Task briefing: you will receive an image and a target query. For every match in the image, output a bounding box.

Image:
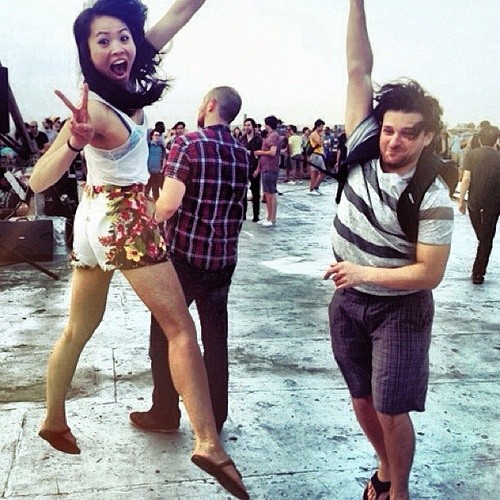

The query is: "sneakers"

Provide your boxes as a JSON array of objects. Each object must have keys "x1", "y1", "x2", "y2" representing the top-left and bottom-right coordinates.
[
  {"x1": 317, "y1": 190, "x2": 323, "y2": 195},
  {"x1": 299, "y1": 180, "x2": 304, "y2": 184},
  {"x1": 307, "y1": 189, "x2": 320, "y2": 196},
  {"x1": 257, "y1": 219, "x2": 268, "y2": 225},
  {"x1": 287, "y1": 181, "x2": 296, "y2": 185},
  {"x1": 262, "y1": 221, "x2": 276, "y2": 227}
]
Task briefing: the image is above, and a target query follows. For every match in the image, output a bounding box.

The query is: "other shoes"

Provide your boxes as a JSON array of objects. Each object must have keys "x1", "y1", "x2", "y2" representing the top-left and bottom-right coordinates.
[
  {"x1": 129, "y1": 412, "x2": 181, "y2": 432},
  {"x1": 473, "y1": 274, "x2": 483, "y2": 284}
]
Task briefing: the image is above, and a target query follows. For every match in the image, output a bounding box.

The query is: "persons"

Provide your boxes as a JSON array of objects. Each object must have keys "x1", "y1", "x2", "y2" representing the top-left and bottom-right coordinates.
[
  {"x1": 131, "y1": 87, "x2": 251, "y2": 435},
  {"x1": 458, "y1": 125, "x2": 499, "y2": 288},
  {"x1": 323, "y1": 1, "x2": 455, "y2": 500},
  {"x1": 0, "y1": 116, "x2": 499, "y2": 222},
  {"x1": 28, "y1": 1, "x2": 250, "y2": 500}
]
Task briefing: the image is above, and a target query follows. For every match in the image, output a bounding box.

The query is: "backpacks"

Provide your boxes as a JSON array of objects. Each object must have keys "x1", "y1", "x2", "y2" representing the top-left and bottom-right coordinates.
[{"x1": 335, "y1": 134, "x2": 459, "y2": 243}]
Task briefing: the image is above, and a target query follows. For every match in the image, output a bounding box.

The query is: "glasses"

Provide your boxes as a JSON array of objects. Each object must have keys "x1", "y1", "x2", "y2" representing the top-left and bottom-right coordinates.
[{"x1": 29, "y1": 126, "x2": 34, "y2": 128}]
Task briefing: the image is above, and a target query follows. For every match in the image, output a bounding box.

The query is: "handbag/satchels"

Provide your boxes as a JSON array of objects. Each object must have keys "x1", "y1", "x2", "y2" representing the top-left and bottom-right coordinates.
[{"x1": 307, "y1": 145, "x2": 319, "y2": 155}]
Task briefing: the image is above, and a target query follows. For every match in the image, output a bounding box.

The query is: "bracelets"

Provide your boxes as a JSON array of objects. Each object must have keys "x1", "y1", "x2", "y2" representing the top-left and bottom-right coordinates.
[{"x1": 65, "y1": 136, "x2": 87, "y2": 158}]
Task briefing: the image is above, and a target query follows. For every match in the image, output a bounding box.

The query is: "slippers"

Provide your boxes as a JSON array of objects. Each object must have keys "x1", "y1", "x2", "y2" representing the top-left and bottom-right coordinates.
[
  {"x1": 39, "y1": 428, "x2": 80, "y2": 453},
  {"x1": 192, "y1": 454, "x2": 250, "y2": 500},
  {"x1": 362, "y1": 472, "x2": 391, "y2": 500}
]
